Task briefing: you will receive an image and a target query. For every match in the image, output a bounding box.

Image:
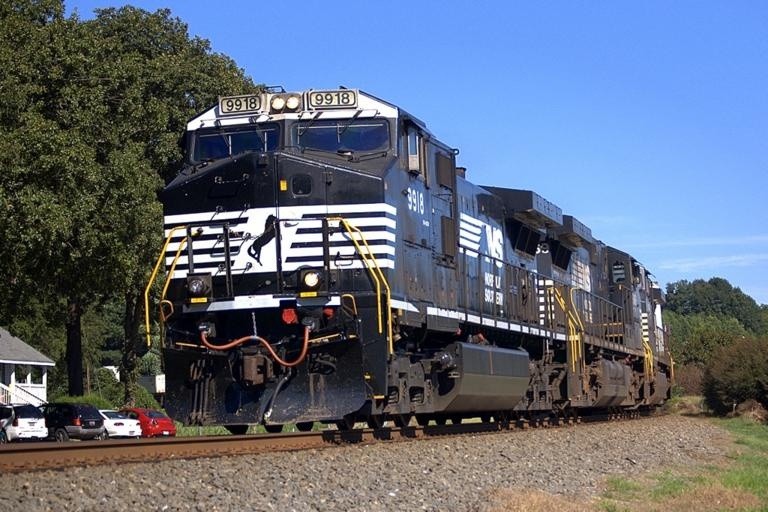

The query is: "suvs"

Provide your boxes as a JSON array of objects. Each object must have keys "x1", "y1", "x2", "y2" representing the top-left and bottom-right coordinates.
[{"x1": 0, "y1": 401, "x2": 106, "y2": 444}]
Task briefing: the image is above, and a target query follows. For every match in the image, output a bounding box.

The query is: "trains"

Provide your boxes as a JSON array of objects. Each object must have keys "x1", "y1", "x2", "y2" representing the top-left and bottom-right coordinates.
[{"x1": 158, "y1": 84, "x2": 676, "y2": 434}]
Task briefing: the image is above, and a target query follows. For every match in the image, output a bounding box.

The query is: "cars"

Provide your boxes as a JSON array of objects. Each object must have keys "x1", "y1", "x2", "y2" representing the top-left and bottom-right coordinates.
[{"x1": 89, "y1": 407, "x2": 177, "y2": 440}]
